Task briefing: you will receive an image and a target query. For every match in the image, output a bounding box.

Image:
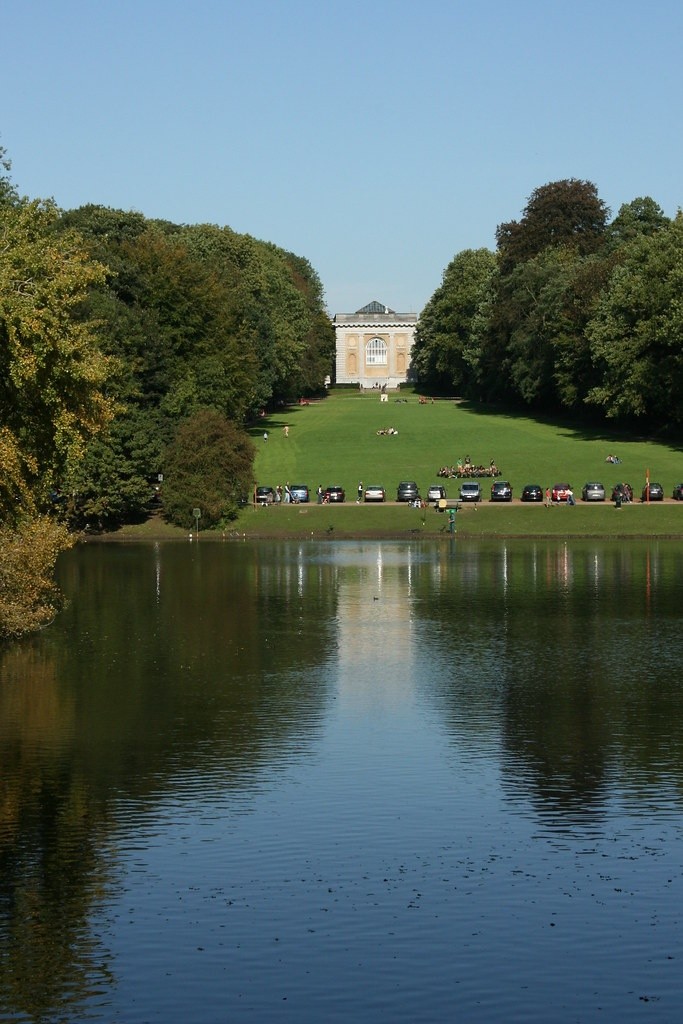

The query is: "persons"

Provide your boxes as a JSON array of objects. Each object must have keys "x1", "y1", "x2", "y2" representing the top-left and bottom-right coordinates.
[
  {"x1": 356, "y1": 482, "x2": 363, "y2": 504},
  {"x1": 556, "y1": 493, "x2": 561, "y2": 503},
  {"x1": 565, "y1": 489, "x2": 575, "y2": 505},
  {"x1": 263, "y1": 433, "x2": 268, "y2": 442},
  {"x1": 395, "y1": 398, "x2": 408, "y2": 403},
  {"x1": 438, "y1": 455, "x2": 502, "y2": 479},
  {"x1": 419, "y1": 396, "x2": 434, "y2": 404},
  {"x1": 545, "y1": 488, "x2": 553, "y2": 507},
  {"x1": 266, "y1": 481, "x2": 300, "y2": 504},
  {"x1": 300, "y1": 396, "x2": 309, "y2": 406},
  {"x1": 377, "y1": 427, "x2": 398, "y2": 436},
  {"x1": 316, "y1": 485, "x2": 327, "y2": 504},
  {"x1": 282, "y1": 426, "x2": 289, "y2": 439},
  {"x1": 420, "y1": 500, "x2": 426, "y2": 521},
  {"x1": 616, "y1": 483, "x2": 631, "y2": 509},
  {"x1": 606, "y1": 455, "x2": 620, "y2": 464},
  {"x1": 448, "y1": 509, "x2": 455, "y2": 535}
]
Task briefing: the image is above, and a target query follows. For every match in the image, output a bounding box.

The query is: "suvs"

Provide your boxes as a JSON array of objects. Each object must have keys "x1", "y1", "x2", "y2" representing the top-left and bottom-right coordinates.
[
  {"x1": 326, "y1": 487, "x2": 344, "y2": 502},
  {"x1": 491, "y1": 482, "x2": 511, "y2": 501},
  {"x1": 552, "y1": 483, "x2": 571, "y2": 501},
  {"x1": 642, "y1": 483, "x2": 663, "y2": 501}
]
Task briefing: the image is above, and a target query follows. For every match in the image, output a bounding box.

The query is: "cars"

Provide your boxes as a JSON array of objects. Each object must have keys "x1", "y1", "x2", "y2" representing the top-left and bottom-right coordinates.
[
  {"x1": 673, "y1": 484, "x2": 683, "y2": 501},
  {"x1": 365, "y1": 485, "x2": 384, "y2": 502},
  {"x1": 254, "y1": 486, "x2": 276, "y2": 502},
  {"x1": 428, "y1": 486, "x2": 445, "y2": 501},
  {"x1": 523, "y1": 485, "x2": 543, "y2": 501},
  {"x1": 612, "y1": 483, "x2": 633, "y2": 501}
]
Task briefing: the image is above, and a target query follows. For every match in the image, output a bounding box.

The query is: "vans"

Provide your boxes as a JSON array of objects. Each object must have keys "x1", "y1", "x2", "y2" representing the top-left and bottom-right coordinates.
[
  {"x1": 583, "y1": 483, "x2": 604, "y2": 501},
  {"x1": 398, "y1": 481, "x2": 418, "y2": 501},
  {"x1": 289, "y1": 485, "x2": 308, "y2": 502},
  {"x1": 460, "y1": 482, "x2": 480, "y2": 501}
]
